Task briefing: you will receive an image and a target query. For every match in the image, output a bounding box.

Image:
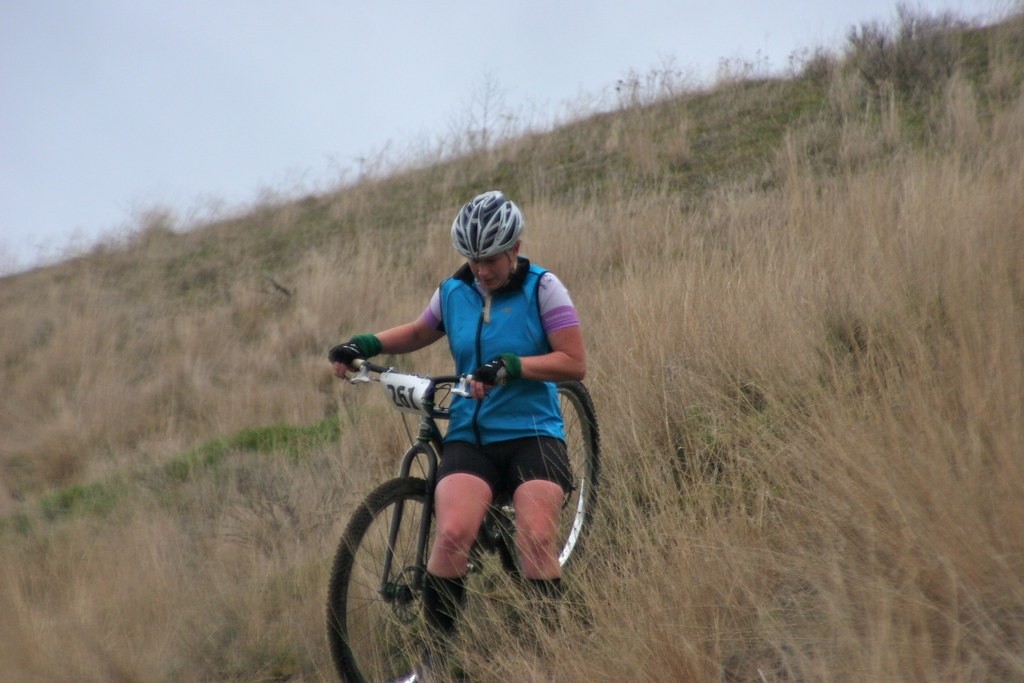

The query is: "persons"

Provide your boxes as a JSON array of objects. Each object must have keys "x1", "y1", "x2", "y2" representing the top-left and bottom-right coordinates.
[{"x1": 328, "y1": 189, "x2": 587, "y2": 683}]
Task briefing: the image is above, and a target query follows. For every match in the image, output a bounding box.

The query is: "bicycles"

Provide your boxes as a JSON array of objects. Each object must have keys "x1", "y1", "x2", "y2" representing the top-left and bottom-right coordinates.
[{"x1": 326, "y1": 358, "x2": 601, "y2": 683}]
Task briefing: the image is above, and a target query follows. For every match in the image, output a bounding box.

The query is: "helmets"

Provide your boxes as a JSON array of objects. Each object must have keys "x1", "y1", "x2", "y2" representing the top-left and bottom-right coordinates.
[{"x1": 451, "y1": 190, "x2": 523, "y2": 260}]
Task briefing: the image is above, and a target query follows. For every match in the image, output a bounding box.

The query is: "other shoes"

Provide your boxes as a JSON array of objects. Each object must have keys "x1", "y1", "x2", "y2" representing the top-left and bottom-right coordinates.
[{"x1": 391, "y1": 664, "x2": 445, "y2": 683}]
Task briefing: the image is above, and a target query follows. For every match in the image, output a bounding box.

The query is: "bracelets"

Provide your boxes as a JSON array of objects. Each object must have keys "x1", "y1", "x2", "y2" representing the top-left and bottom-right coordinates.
[
  {"x1": 349, "y1": 333, "x2": 382, "y2": 358},
  {"x1": 500, "y1": 353, "x2": 521, "y2": 381}
]
